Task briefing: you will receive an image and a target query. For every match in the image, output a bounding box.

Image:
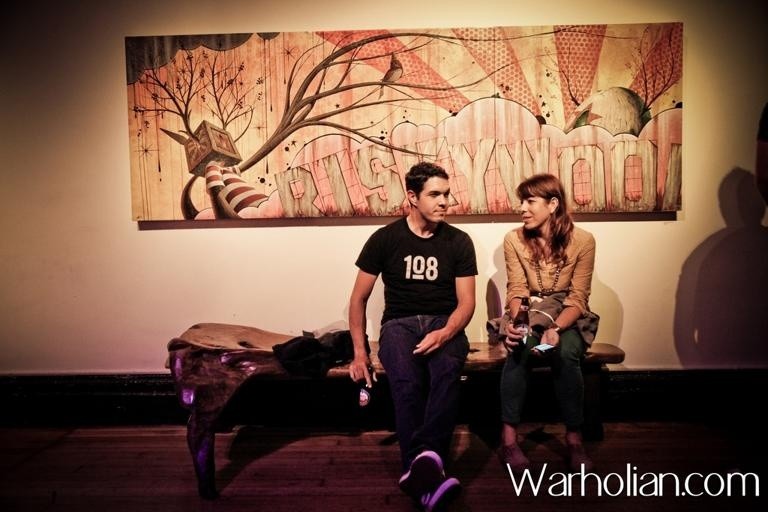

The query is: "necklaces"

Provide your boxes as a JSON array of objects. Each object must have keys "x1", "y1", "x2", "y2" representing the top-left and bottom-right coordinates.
[{"x1": 533, "y1": 255, "x2": 561, "y2": 293}]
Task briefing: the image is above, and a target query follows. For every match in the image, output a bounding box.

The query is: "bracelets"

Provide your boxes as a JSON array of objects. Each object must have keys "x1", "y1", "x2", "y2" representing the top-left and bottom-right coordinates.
[{"x1": 548, "y1": 323, "x2": 562, "y2": 335}]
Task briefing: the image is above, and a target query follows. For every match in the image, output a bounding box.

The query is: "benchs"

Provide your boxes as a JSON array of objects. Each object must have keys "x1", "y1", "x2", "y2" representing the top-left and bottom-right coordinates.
[{"x1": 167, "y1": 321, "x2": 625, "y2": 501}]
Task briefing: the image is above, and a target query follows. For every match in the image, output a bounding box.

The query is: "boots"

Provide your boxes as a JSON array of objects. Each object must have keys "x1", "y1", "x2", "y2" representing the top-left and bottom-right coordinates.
[
  {"x1": 568, "y1": 443, "x2": 593, "y2": 471},
  {"x1": 496, "y1": 441, "x2": 531, "y2": 473}
]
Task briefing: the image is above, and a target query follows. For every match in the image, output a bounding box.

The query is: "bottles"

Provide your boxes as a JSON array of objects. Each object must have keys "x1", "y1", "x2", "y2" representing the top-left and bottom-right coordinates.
[
  {"x1": 510, "y1": 297, "x2": 530, "y2": 365},
  {"x1": 359, "y1": 363, "x2": 375, "y2": 409}
]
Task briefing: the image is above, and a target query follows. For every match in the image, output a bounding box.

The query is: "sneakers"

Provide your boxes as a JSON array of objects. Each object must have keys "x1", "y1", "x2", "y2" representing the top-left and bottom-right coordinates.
[{"x1": 397, "y1": 451, "x2": 460, "y2": 512}]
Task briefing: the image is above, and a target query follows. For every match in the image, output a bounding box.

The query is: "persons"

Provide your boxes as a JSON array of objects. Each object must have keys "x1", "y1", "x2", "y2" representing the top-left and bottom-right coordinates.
[
  {"x1": 497, "y1": 173, "x2": 605, "y2": 486},
  {"x1": 347, "y1": 162, "x2": 479, "y2": 509}
]
